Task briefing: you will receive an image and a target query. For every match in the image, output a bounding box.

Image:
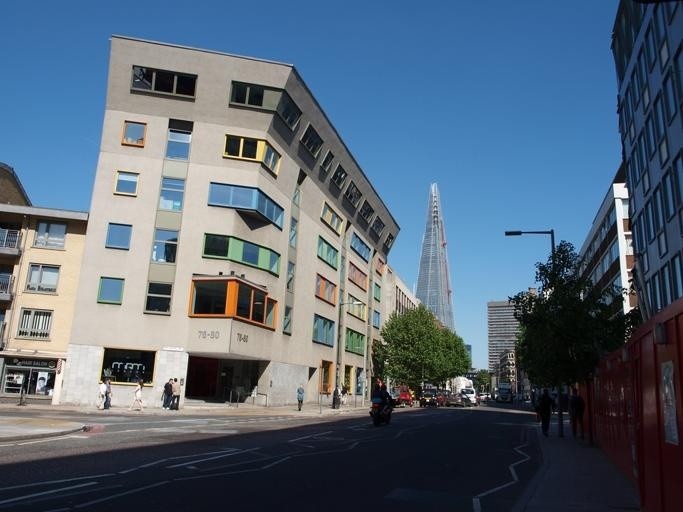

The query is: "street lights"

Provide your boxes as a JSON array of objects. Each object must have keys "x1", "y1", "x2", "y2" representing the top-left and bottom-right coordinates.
[
  {"x1": 332, "y1": 301, "x2": 364, "y2": 409},
  {"x1": 504, "y1": 226, "x2": 566, "y2": 436}
]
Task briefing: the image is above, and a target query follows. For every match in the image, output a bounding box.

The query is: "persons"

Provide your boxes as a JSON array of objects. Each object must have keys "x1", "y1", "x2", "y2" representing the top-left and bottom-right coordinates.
[
  {"x1": 334, "y1": 384, "x2": 341, "y2": 409},
  {"x1": 537, "y1": 389, "x2": 556, "y2": 438},
  {"x1": 169, "y1": 378, "x2": 181, "y2": 410},
  {"x1": 372, "y1": 382, "x2": 392, "y2": 414},
  {"x1": 36, "y1": 377, "x2": 47, "y2": 396},
  {"x1": 567, "y1": 388, "x2": 585, "y2": 439},
  {"x1": 340, "y1": 385, "x2": 349, "y2": 405},
  {"x1": 129, "y1": 380, "x2": 146, "y2": 412},
  {"x1": 160, "y1": 378, "x2": 174, "y2": 410},
  {"x1": 95, "y1": 376, "x2": 114, "y2": 410},
  {"x1": 297, "y1": 383, "x2": 304, "y2": 411}
]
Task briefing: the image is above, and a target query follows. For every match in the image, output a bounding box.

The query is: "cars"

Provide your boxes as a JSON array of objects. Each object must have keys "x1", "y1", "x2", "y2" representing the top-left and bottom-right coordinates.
[{"x1": 391, "y1": 383, "x2": 530, "y2": 410}]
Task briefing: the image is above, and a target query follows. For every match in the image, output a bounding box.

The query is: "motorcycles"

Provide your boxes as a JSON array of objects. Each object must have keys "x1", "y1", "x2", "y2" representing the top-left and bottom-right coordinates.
[{"x1": 369, "y1": 397, "x2": 394, "y2": 425}]
